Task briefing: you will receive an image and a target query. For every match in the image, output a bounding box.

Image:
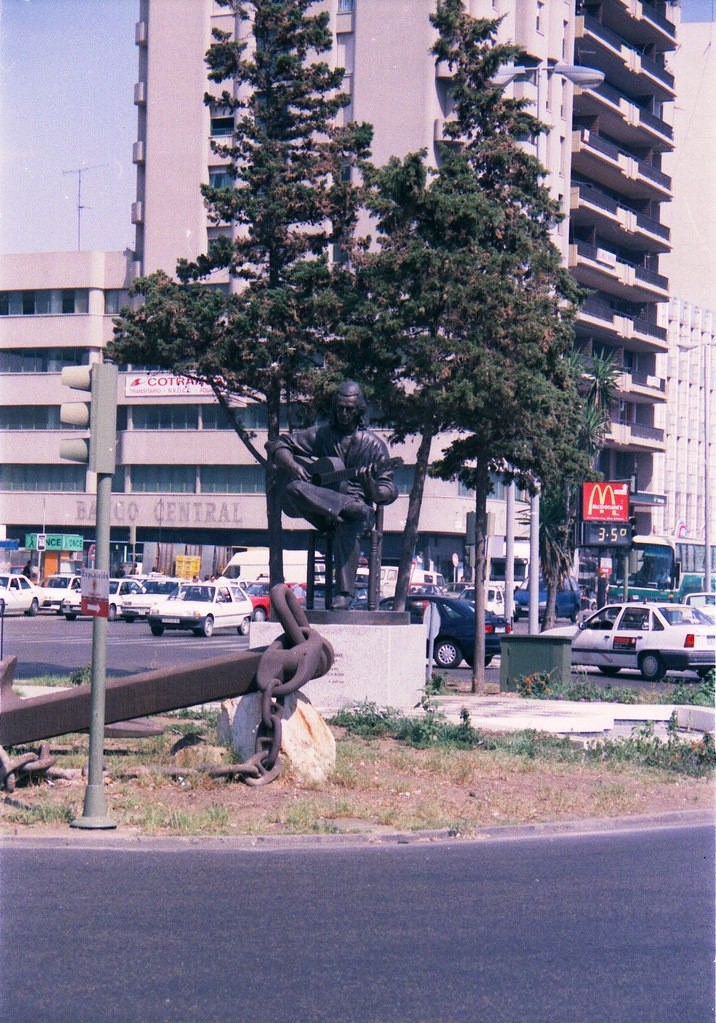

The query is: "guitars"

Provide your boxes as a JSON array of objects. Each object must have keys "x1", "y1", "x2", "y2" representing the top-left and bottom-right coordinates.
[{"x1": 277, "y1": 455, "x2": 405, "y2": 519}]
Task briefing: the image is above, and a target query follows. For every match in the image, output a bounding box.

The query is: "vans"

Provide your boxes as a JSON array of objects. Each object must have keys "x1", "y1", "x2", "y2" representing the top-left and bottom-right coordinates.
[{"x1": 220, "y1": 548, "x2": 326, "y2": 584}]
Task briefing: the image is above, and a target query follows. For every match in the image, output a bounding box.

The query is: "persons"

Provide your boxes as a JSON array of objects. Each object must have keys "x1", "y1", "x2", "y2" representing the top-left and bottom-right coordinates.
[
  {"x1": 580, "y1": 586, "x2": 594, "y2": 610},
  {"x1": 149, "y1": 567, "x2": 158, "y2": 577},
  {"x1": 460, "y1": 577, "x2": 465, "y2": 582},
  {"x1": 264, "y1": 382, "x2": 399, "y2": 610},
  {"x1": 76, "y1": 563, "x2": 86, "y2": 576},
  {"x1": 190, "y1": 573, "x2": 230, "y2": 601},
  {"x1": 23, "y1": 561, "x2": 31, "y2": 579},
  {"x1": 128, "y1": 563, "x2": 139, "y2": 575},
  {"x1": 656, "y1": 570, "x2": 670, "y2": 584},
  {"x1": 114, "y1": 567, "x2": 125, "y2": 578}
]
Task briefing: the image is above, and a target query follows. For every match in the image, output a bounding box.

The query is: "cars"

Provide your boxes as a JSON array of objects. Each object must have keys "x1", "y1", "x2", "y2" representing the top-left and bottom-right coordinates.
[
  {"x1": 147, "y1": 582, "x2": 253, "y2": 637},
  {"x1": 309, "y1": 581, "x2": 383, "y2": 611},
  {"x1": 680, "y1": 592, "x2": 716, "y2": 620},
  {"x1": 0, "y1": 574, "x2": 45, "y2": 618},
  {"x1": 63, "y1": 572, "x2": 203, "y2": 623},
  {"x1": 539, "y1": 599, "x2": 716, "y2": 682},
  {"x1": 341, "y1": 563, "x2": 587, "y2": 669},
  {"x1": 121, "y1": 578, "x2": 187, "y2": 624},
  {"x1": 244, "y1": 581, "x2": 309, "y2": 623},
  {"x1": 36, "y1": 572, "x2": 78, "y2": 617}
]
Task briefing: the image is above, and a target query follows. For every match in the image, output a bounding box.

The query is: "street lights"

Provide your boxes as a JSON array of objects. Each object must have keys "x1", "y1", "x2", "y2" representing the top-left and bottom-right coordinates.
[{"x1": 677, "y1": 340, "x2": 716, "y2": 592}]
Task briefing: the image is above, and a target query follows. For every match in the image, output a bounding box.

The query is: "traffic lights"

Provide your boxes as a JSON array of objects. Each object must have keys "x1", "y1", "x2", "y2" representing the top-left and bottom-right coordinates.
[{"x1": 58, "y1": 359, "x2": 117, "y2": 475}]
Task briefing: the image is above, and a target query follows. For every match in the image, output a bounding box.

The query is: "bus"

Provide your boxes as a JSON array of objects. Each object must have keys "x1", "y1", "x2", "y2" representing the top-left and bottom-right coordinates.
[{"x1": 603, "y1": 535, "x2": 716, "y2": 612}]
